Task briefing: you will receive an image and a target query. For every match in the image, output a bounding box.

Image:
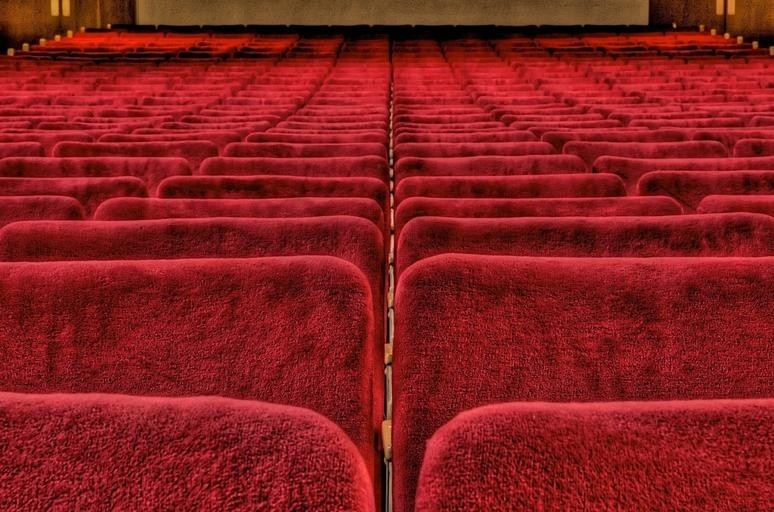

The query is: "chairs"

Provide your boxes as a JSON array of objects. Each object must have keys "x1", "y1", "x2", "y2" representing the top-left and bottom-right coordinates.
[{"x1": 0, "y1": 22, "x2": 773, "y2": 512}]
